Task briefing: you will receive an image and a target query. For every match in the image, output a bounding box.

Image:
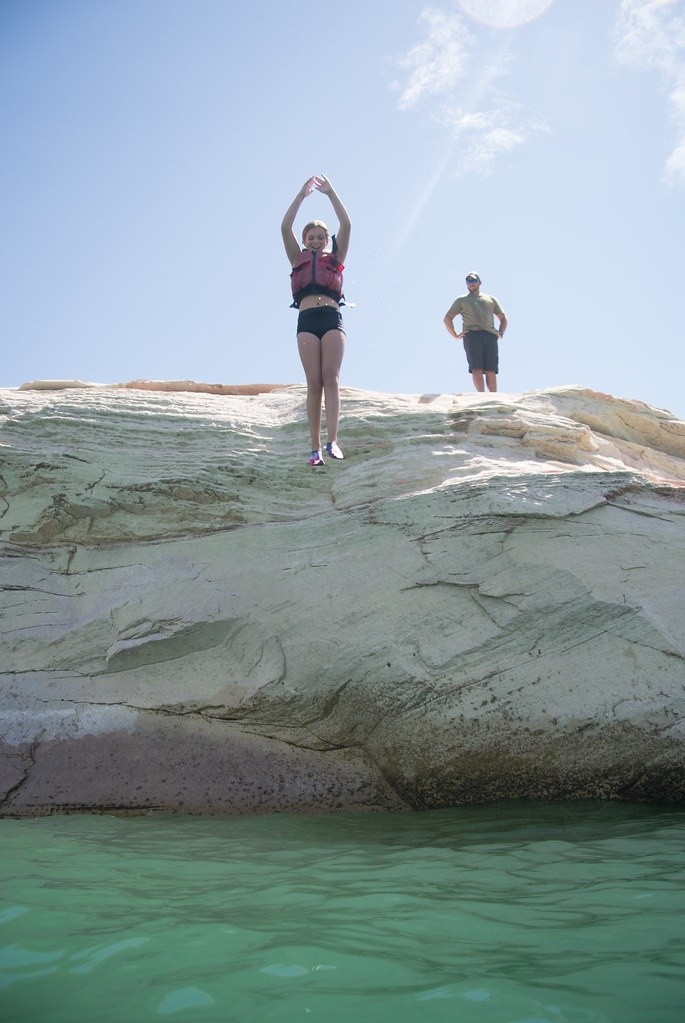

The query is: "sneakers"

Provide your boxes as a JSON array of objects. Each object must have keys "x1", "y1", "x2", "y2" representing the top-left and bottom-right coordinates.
[
  {"x1": 310, "y1": 450, "x2": 324, "y2": 465},
  {"x1": 327, "y1": 443, "x2": 344, "y2": 460}
]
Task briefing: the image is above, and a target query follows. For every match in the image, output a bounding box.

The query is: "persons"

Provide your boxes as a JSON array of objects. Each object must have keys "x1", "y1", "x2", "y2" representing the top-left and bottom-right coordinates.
[
  {"x1": 281, "y1": 172, "x2": 352, "y2": 470},
  {"x1": 444, "y1": 271, "x2": 507, "y2": 392}
]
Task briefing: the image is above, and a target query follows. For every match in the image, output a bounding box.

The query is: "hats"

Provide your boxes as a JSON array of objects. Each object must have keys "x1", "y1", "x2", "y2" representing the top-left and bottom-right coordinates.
[{"x1": 466, "y1": 273, "x2": 481, "y2": 283}]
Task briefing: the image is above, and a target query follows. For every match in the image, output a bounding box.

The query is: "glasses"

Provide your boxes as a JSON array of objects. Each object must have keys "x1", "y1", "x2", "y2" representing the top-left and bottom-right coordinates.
[{"x1": 466, "y1": 279, "x2": 478, "y2": 284}]
